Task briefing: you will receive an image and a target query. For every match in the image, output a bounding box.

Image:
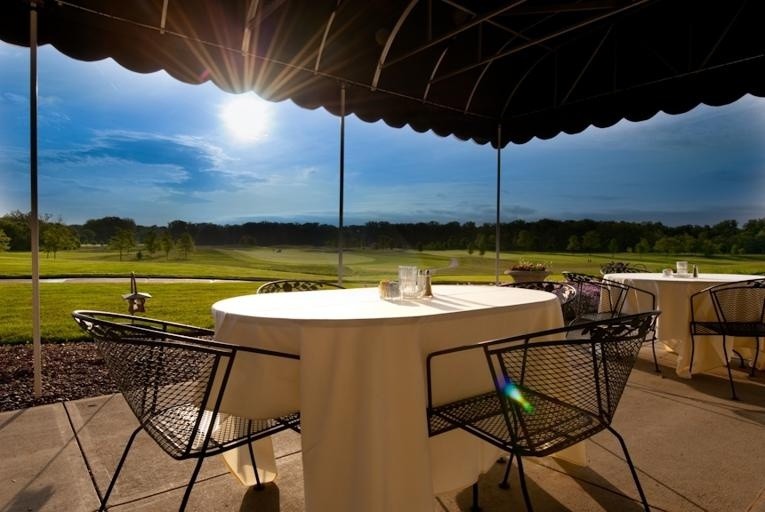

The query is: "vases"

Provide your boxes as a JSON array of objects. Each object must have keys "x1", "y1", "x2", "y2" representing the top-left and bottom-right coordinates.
[{"x1": 507, "y1": 267, "x2": 553, "y2": 282}]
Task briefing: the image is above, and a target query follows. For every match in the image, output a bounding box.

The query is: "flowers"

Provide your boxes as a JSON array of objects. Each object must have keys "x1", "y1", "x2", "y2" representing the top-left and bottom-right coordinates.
[{"x1": 509, "y1": 257, "x2": 555, "y2": 272}]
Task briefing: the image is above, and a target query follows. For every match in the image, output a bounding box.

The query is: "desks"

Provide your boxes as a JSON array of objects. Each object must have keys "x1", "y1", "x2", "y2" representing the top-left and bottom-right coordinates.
[
  {"x1": 207, "y1": 285, "x2": 565, "y2": 512},
  {"x1": 598, "y1": 272, "x2": 765, "y2": 378}
]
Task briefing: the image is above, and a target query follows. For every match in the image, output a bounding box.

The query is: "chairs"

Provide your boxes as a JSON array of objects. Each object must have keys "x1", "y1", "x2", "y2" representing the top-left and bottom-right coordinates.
[
  {"x1": 683, "y1": 278, "x2": 765, "y2": 402},
  {"x1": 564, "y1": 270, "x2": 662, "y2": 373},
  {"x1": 599, "y1": 265, "x2": 640, "y2": 276},
  {"x1": 504, "y1": 280, "x2": 579, "y2": 306},
  {"x1": 64, "y1": 304, "x2": 301, "y2": 509},
  {"x1": 422, "y1": 309, "x2": 660, "y2": 510},
  {"x1": 250, "y1": 279, "x2": 351, "y2": 491}
]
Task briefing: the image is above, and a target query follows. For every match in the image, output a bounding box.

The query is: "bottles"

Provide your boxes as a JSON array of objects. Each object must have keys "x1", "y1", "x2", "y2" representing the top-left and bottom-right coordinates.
[
  {"x1": 693, "y1": 264, "x2": 698, "y2": 277},
  {"x1": 416, "y1": 269, "x2": 425, "y2": 298},
  {"x1": 422, "y1": 270, "x2": 433, "y2": 299}
]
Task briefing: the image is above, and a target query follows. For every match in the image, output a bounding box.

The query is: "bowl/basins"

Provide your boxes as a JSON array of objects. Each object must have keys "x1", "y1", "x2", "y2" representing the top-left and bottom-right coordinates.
[{"x1": 662, "y1": 269, "x2": 674, "y2": 276}]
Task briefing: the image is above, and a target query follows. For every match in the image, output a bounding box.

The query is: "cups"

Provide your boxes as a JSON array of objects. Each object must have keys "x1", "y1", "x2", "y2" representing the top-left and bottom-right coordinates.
[
  {"x1": 676, "y1": 261, "x2": 687, "y2": 277},
  {"x1": 398, "y1": 265, "x2": 418, "y2": 299}
]
